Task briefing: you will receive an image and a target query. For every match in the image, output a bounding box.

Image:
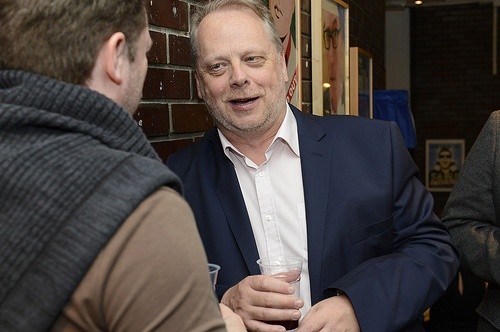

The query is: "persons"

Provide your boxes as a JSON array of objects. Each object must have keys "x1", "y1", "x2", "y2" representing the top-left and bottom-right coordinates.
[
  {"x1": 159, "y1": 0, "x2": 460, "y2": 332},
  {"x1": 443, "y1": 110, "x2": 500, "y2": 331},
  {"x1": 320, "y1": 11, "x2": 346, "y2": 115},
  {"x1": 0, "y1": 1, "x2": 247, "y2": 332}
]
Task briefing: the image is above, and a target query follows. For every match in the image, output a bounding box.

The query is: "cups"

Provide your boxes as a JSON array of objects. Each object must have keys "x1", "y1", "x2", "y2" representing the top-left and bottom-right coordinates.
[
  {"x1": 257, "y1": 256, "x2": 304, "y2": 300},
  {"x1": 208, "y1": 261, "x2": 220, "y2": 294}
]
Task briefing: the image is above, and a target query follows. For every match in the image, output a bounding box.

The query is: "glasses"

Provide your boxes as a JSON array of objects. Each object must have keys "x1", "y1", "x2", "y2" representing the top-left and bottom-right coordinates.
[{"x1": 438, "y1": 155, "x2": 451, "y2": 159}]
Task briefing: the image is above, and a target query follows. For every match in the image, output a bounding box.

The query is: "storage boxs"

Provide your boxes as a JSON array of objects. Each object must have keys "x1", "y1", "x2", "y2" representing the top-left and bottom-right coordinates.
[{"x1": 424, "y1": 139, "x2": 465, "y2": 193}]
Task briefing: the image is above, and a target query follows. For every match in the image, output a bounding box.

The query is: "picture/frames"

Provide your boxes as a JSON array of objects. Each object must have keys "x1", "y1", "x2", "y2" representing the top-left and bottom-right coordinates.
[
  {"x1": 261, "y1": 0, "x2": 303, "y2": 111},
  {"x1": 349, "y1": 47, "x2": 373, "y2": 119},
  {"x1": 311, "y1": 0, "x2": 350, "y2": 117}
]
